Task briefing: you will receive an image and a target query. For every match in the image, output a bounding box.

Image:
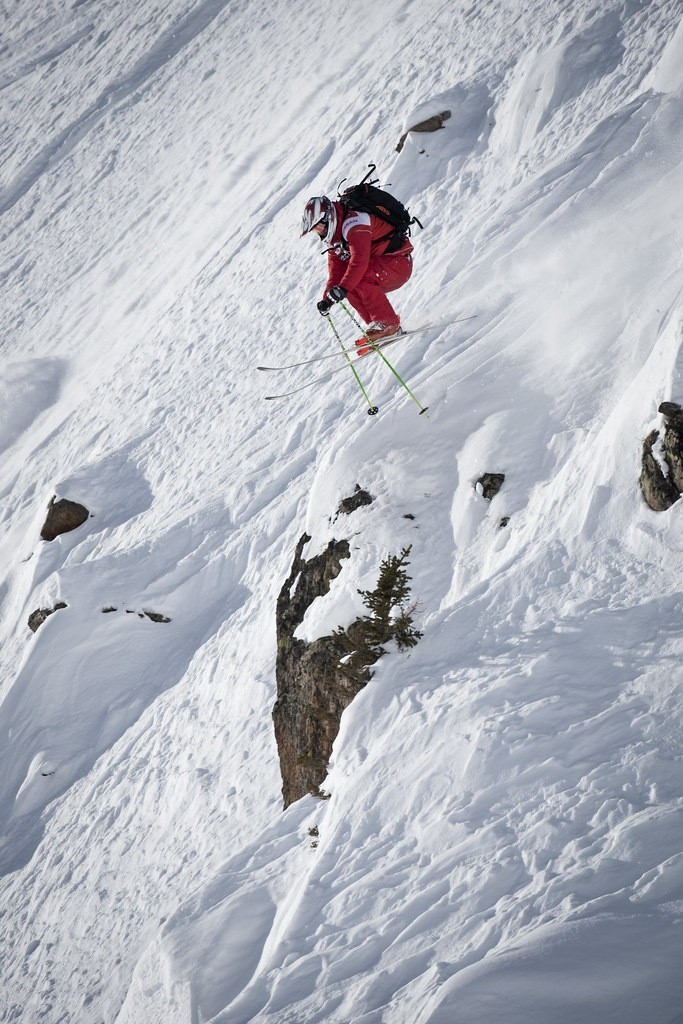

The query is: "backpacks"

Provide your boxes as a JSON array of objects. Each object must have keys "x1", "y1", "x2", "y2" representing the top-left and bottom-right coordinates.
[{"x1": 340, "y1": 184, "x2": 411, "y2": 238}]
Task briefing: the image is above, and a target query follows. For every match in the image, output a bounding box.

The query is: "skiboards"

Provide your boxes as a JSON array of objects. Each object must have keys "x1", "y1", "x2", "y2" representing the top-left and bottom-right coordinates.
[{"x1": 256, "y1": 315, "x2": 478, "y2": 401}]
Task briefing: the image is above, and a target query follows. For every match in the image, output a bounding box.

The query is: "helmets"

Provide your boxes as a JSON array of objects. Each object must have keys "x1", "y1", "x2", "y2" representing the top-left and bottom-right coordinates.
[{"x1": 300, "y1": 196, "x2": 330, "y2": 237}]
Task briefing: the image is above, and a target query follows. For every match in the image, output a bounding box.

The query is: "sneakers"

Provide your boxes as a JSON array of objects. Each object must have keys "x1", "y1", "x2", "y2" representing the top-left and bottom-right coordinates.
[{"x1": 354, "y1": 318, "x2": 404, "y2": 358}]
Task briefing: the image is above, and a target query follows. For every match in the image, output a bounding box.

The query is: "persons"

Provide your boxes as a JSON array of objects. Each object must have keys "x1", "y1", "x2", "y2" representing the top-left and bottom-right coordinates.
[{"x1": 295, "y1": 193, "x2": 416, "y2": 355}]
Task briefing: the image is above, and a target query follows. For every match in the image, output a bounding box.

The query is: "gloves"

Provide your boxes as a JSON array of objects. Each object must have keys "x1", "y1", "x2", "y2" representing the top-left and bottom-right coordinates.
[
  {"x1": 327, "y1": 285, "x2": 347, "y2": 304},
  {"x1": 317, "y1": 299, "x2": 333, "y2": 316}
]
